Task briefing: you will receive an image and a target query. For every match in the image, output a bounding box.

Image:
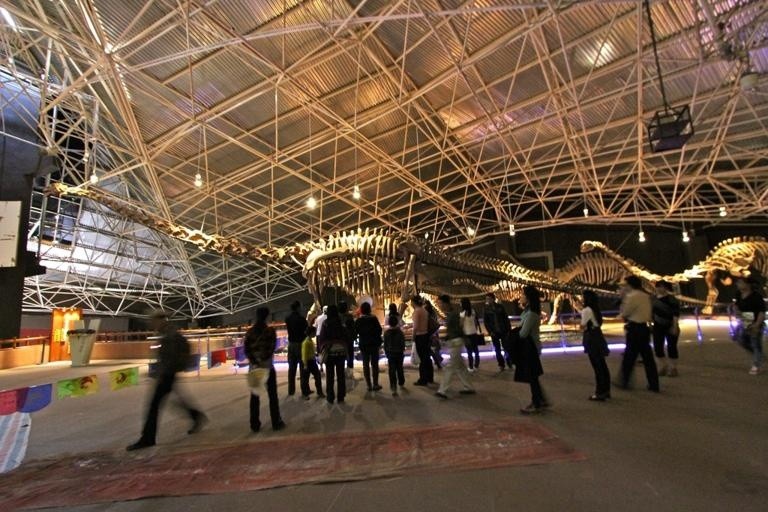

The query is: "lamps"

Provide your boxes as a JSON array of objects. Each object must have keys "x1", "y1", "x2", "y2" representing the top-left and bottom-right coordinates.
[
  {"x1": 192, "y1": 122, "x2": 209, "y2": 189},
  {"x1": 305, "y1": 109, "x2": 317, "y2": 211},
  {"x1": 351, "y1": 41, "x2": 363, "y2": 202},
  {"x1": 679, "y1": 208, "x2": 690, "y2": 242},
  {"x1": 636, "y1": 193, "x2": 647, "y2": 245},
  {"x1": 463, "y1": 216, "x2": 477, "y2": 239},
  {"x1": 500, "y1": 131, "x2": 516, "y2": 239},
  {"x1": 87, "y1": 96, "x2": 100, "y2": 186},
  {"x1": 581, "y1": 158, "x2": 590, "y2": 219}
]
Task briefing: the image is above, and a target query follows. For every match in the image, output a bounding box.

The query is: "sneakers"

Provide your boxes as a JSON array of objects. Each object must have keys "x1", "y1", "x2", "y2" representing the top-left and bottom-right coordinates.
[
  {"x1": 588, "y1": 394, "x2": 608, "y2": 402},
  {"x1": 458, "y1": 389, "x2": 477, "y2": 395},
  {"x1": 126, "y1": 435, "x2": 156, "y2": 451},
  {"x1": 658, "y1": 366, "x2": 678, "y2": 377},
  {"x1": 433, "y1": 391, "x2": 448, "y2": 400},
  {"x1": 748, "y1": 365, "x2": 768, "y2": 375},
  {"x1": 520, "y1": 404, "x2": 545, "y2": 414}
]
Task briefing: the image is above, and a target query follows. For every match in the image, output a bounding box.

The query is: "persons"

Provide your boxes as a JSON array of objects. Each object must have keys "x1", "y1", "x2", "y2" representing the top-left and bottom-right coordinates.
[
  {"x1": 125, "y1": 310, "x2": 209, "y2": 452},
  {"x1": 243, "y1": 307, "x2": 285, "y2": 432},
  {"x1": 732, "y1": 278, "x2": 767, "y2": 376}
]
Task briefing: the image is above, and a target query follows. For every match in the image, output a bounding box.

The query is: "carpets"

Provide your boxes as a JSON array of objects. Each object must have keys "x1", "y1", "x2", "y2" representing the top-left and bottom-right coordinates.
[{"x1": 1, "y1": 415, "x2": 593, "y2": 510}]
